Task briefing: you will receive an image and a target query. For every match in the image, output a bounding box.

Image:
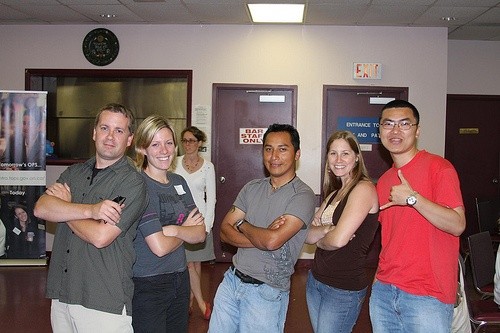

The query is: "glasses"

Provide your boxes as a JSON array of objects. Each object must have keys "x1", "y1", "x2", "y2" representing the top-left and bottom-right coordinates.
[
  {"x1": 180, "y1": 139, "x2": 199, "y2": 144},
  {"x1": 103, "y1": 195, "x2": 126, "y2": 224},
  {"x1": 380, "y1": 121, "x2": 418, "y2": 130}
]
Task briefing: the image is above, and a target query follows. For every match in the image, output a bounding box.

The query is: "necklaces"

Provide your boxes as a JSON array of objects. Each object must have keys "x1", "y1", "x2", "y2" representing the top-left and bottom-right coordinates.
[
  {"x1": 183, "y1": 158, "x2": 200, "y2": 171},
  {"x1": 269, "y1": 173, "x2": 296, "y2": 189}
]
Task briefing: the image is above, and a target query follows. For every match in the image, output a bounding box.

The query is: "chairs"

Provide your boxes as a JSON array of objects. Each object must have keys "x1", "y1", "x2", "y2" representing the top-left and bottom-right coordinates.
[{"x1": 461, "y1": 196, "x2": 500, "y2": 333}]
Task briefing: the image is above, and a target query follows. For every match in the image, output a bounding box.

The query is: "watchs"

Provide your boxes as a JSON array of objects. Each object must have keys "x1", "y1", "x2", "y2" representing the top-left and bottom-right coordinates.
[
  {"x1": 406, "y1": 191, "x2": 418, "y2": 206},
  {"x1": 236, "y1": 218, "x2": 247, "y2": 231}
]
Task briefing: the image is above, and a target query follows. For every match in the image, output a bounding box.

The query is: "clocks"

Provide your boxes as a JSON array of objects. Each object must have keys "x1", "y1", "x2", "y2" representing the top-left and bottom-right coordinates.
[{"x1": 83, "y1": 28, "x2": 120, "y2": 66}]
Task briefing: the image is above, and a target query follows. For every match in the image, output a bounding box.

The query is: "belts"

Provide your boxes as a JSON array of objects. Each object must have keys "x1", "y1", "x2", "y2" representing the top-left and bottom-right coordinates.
[{"x1": 231, "y1": 264, "x2": 264, "y2": 284}]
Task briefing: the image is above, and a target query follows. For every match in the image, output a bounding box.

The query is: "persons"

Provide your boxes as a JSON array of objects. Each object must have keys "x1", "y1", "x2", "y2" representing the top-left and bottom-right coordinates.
[
  {"x1": 304, "y1": 130, "x2": 383, "y2": 333},
  {"x1": 172, "y1": 127, "x2": 217, "y2": 320},
  {"x1": 6, "y1": 206, "x2": 39, "y2": 255},
  {"x1": 4, "y1": 106, "x2": 42, "y2": 165},
  {"x1": 130, "y1": 114, "x2": 206, "y2": 333},
  {"x1": 206, "y1": 123, "x2": 315, "y2": 333},
  {"x1": 369, "y1": 101, "x2": 466, "y2": 333},
  {"x1": 494, "y1": 245, "x2": 500, "y2": 305},
  {"x1": 34, "y1": 104, "x2": 150, "y2": 333}
]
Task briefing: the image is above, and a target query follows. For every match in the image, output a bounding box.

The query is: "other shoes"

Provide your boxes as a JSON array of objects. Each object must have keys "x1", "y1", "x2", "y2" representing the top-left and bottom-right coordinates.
[{"x1": 204, "y1": 303, "x2": 212, "y2": 320}]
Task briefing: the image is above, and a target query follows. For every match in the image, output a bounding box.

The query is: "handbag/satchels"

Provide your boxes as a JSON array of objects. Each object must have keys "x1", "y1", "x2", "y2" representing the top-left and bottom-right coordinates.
[{"x1": 183, "y1": 232, "x2": 208, "y2": 251}]
